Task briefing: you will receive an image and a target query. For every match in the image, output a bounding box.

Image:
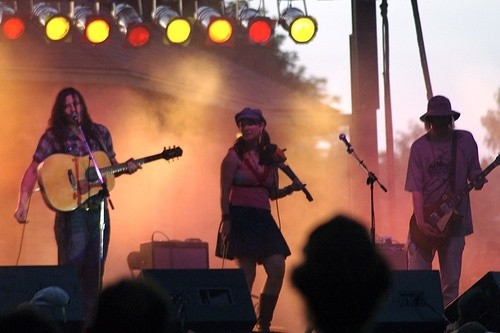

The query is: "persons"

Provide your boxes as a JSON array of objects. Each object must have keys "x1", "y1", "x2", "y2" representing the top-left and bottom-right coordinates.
[
  {"x1": 220, "y1": 107, "x2": 306, "y2": 333},
  {"x1": 0, "y1": 286, "x2": 84, "y2": 333},
  {"x1": 292, "y1": 216, "x2": 390, "y2": 333},
  {"x1": 82, "y1": 278, "x2": 181, "y2": 333},
  {"x1": 404, "y1": 96, "x2": 487, "y2": 309},
  {"x1": 447, "y1": 285, "x2": 500, "y2": 333},
  {"x1": 14, "y1": 87, "x2": 142, "y2": 314}
]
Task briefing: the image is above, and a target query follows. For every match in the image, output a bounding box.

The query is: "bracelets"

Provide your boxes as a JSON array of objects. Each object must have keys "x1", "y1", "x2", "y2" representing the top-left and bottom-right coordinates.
[
  {"x1": 222, "y1": 214, "x2": 230, "y2": 220},
  {"x1": 285, "y1": 185, "x2": 293, "y2": 195}
]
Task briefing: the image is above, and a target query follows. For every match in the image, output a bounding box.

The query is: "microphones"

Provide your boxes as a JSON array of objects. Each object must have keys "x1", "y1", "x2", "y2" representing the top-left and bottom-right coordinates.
[
  {"x1": 71, "y1": 112, "x2": 78, "y2": 120},
  {"x1": 338, "y1": 134, "x2": 353, "y2": 152}
]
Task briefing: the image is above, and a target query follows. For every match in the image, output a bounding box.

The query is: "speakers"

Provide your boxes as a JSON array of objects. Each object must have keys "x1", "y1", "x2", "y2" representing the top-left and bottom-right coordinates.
[
  {"x1": 366, "y1": 243, "x2": 500, "y2": 333},
  {"x1": 137, "y1": 241, "x2": 257, "y2": 333},
  {"x1": 0, "y1": 264, "x2": 58, "y2": 316}
]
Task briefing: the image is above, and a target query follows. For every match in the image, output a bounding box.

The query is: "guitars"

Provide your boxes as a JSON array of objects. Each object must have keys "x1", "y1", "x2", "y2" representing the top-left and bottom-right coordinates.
[
  {"x1": 35, "y1": 145, "x2": 184, "y2": 214},
  {"x1": 409, "y1": 152, "x2": 500, "y2": 252}
]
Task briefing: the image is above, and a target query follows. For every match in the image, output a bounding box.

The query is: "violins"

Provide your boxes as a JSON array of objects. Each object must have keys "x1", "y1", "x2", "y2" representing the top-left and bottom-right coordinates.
[{"x1": 258, "y1": 128, "x2": 314, "y2": 202}]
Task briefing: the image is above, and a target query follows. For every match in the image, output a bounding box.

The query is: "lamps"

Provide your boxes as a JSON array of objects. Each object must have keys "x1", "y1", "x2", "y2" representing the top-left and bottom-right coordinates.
[
  {"x1": 277, "y1": 0, "x2": 318, "y2": 44},
  {"x1": 232, "y1": 0, "x2": 279, "y2": 47},
  {"x1": 150, "y1": 0, "x2": 195, "y2": 48},
  {"x1": 30, "y1": 0, "x2": 76, "y2": 44},
  {"x1": 0, "y1": 0, "x2": 27, "y2": 41},
  {"x1": 68, "y1": 0, "x2": 115, "y2": 47},
  {"x1": 109, "y1": 0, "x2": 154, "y2": 50},
  {"x1": 193, "y1": 0, "x2": 238, "y2": 48}
]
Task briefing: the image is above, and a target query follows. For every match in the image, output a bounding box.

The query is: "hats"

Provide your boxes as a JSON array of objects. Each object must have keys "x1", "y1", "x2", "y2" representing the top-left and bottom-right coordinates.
[
  {"x1": 235, "y1": 107, "x2": 266, "y2": 131},
  {"x1": 420, "y1": 96, "x2": 460, "y2": 122}
]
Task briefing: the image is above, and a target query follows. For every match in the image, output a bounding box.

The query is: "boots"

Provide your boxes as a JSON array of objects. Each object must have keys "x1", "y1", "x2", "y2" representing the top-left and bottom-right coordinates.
[{"x1": 252, "y1": 294, "x2": 279, "y2": 333}]
